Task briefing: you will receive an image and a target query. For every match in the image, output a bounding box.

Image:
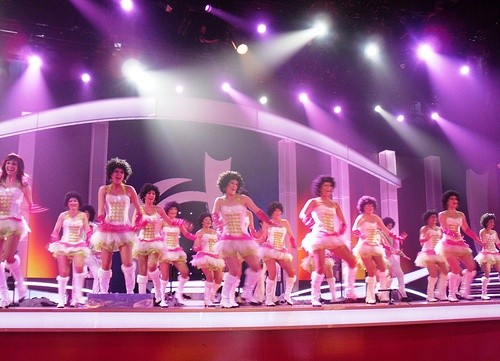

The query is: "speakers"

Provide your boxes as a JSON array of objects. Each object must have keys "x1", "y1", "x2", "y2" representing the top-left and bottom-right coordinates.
[{"x1": 95, "y1": 296, "x2": 155, "y2": 310}]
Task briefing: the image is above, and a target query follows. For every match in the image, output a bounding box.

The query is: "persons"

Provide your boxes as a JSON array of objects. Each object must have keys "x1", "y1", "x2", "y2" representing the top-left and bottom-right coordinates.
[
  {"x1": 473, "y1": 213, "x2": 500, "y2": 300},
  {"x1": 413, "y1": 210, "x2": 449, "y2": 302},
  {"x1": 298, "y1": 174, "x2": 359, "y2": 307},
  {"x1": 132, "y1": 182, "x2": 185, "y2": 308},
  {"x1": 158, "y1": 200, "x2": 198, "y2": 306},
  {"x1": 434, "y1": 190, "x2": 490, "y2": 302},
  {"x1": 376, "y1": 217, "x2": 413, "y2": 302},
  {"x1": 213, "y1": 170, "x2": 299, "y2": 309},
  {"x1": 46, "y1": 190, "x2": 95, "y2": 308},
  {"x1": 352, "y1": 195, "x2": 404, "y2": 304},
  {"x1": 191, "y1": 212, "x2": 227, "y2": 308},
  {"x1": 0, "y1": 152, "x2": 33, "y2": 310},
  {"x1": 90, "y1": 156, "x2": 143, "y2": 294}
]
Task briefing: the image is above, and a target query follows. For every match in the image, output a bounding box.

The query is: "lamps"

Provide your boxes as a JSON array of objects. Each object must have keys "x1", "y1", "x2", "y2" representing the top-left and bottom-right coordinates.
[{"x1": 231, "y1": 37, "x2": 249, "y2": 55}]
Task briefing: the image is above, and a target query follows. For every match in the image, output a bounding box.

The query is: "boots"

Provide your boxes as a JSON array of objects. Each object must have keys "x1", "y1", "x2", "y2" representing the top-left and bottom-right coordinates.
[
  {"x1": 56, "y1": 275, "x2": 70, "y2": 308},
  {"x1": 310, "y1": 271, "x2": 325, "y2": 307},
  {"x1": 396, "y1": 275, "x2": 409, "y2": 301},
  {"x1": 159, "y1": 278, "x2": 169, "y2": 308},
  {"x1": 427, "y1": 268, "x2": 500, "y2": 304},
  {"x1": 70, "y1": 273, "x2": 85, "y2": 307},
  {"x1": 346, "y1": 267, "x2": 359, "y2": 302},
  {"x1": 147, "y1": 268, "x2": 162, "y2": 305},
  {"x1": 5, "y1": 255, "x2": 27, "y2": 302},
  {"x1": 240, "y1": 267, "x2": 260, "y2": 306},
  {"x1": 325, "y1": 277, "x2": 338, "y2": 304},
  {"x1": 264, "y1": 277, "x2": 276, "y2": 307},
  {"x1": 98, "y1": 268, "x2": 112, "y2": 294},
  {"x1": 365, "y1": 276, "x2": 376, "y2": 304},
  {"x1": 377, "y1": 272, "x2": 390, "y2": 302},
  {"x1": 0, "y1": 260, "x2": 10, "y2": 309},
  {"x1": 121, "y1": 261, "x2": 137, "y2": 294},
  {"x1": 205, "y1": 281, "x2": 214, "y2": 307},
  {"x1": 136, "y1": 273, "x2": 149, "y2": 294},
  {"x1": 283, "y1": 275, "x2": 297, "y2": 306},
  {"x1": 228, "y1": 278, "x2": 240, "y2": 308},
  {"x1": 213, "y1": 283, "x2": 221, "y2": 305},
  {"x1": 220, "y1": 272, "x2": 237, "y2": 308},
  {"x1": 175, "y1": 276, "x2": 190, "y2": 304}
]
